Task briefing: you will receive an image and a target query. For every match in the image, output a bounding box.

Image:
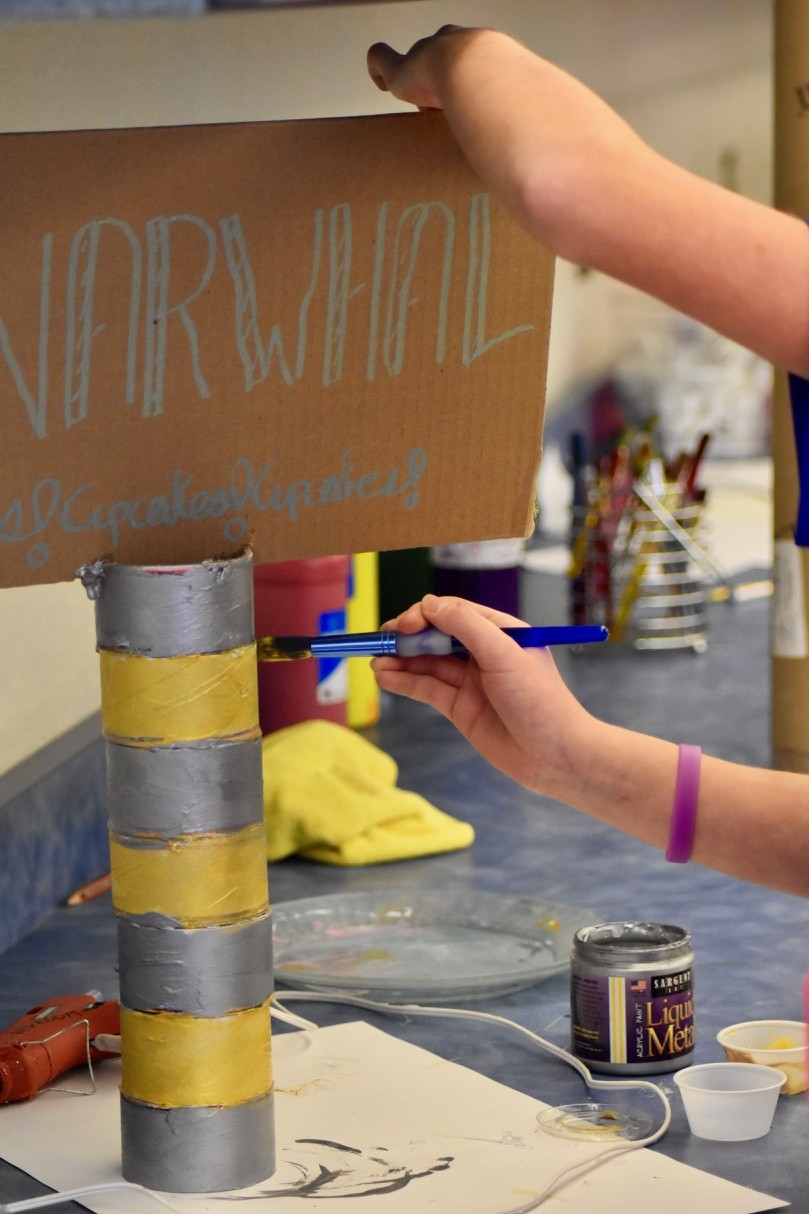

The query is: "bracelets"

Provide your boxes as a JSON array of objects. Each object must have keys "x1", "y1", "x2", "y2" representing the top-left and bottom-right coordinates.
[{"x1": 665, "y1": 744, "x2": 701, "y2": 862}]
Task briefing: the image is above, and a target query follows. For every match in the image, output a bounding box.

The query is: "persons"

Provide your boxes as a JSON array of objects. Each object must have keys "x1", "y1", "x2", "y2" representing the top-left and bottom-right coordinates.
[{"x1": 367, "y1": 22, "x2": 809, "y2": 902}]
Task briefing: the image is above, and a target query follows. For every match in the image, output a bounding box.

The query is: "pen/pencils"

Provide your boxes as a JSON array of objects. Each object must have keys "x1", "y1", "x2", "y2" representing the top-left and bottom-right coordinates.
[
  {"x1": 69, "y1": 873, "x2": 110, "y2": 907},
  {"x1": 564, "y1": 392, "x2": 738, "y2": 659}
]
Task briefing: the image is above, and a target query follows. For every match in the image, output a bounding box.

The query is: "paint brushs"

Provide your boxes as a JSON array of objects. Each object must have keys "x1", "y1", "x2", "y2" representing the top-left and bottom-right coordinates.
[{"x1": 254, "y1": 622, "x2": 607, "y2": 667}]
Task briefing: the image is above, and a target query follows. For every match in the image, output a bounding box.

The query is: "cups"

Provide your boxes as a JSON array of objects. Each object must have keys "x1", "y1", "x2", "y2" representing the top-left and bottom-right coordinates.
[
  {"x1": 430, "y1": 563, "x2": 520, "y2": 620},
  {"x1": 716, "y1": 1019, "x2": 809, "y2": 1095},
  {"x1": 672, "y1": 1062, "x2": 787, "y2": 1141},
  {"x1": 252, "y1": 555, "x2": 353, "y2": 734}
]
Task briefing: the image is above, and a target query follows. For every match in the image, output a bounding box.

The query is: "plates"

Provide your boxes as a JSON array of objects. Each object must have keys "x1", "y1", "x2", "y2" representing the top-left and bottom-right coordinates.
[{"x1": 271, "y1": 888, "x2": 607, "y2": 1003}]
